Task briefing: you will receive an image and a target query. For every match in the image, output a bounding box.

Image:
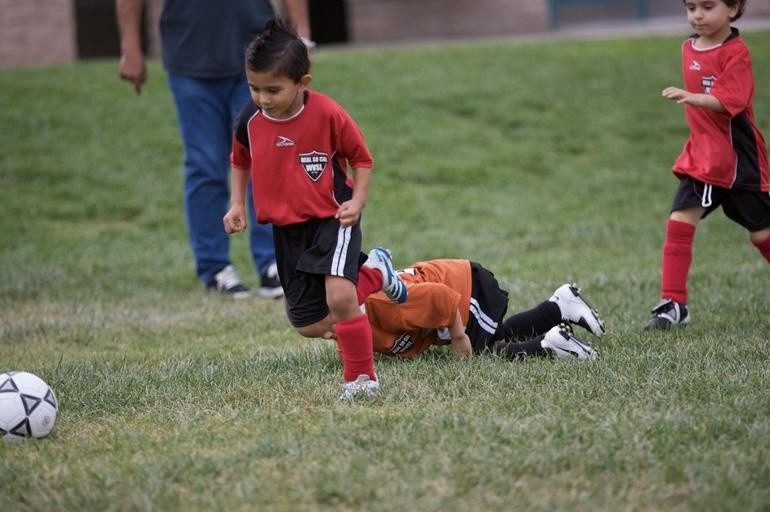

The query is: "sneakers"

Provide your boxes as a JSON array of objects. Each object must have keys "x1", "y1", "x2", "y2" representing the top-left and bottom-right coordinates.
[
  {"x1": 363, "y1": 247, "x2": 408, "y2": 304},
  {"x1": 207, "y1": 264, "x2": 252, "y2": 299},
  {"x1": 644, "y1": 299, "x2": 689, "y2": 331},
  {"x1": 338, "y1": 374, "x2": 379, "y2": 403},
  {"x1": 260, "y1": 260, "x2": 284, "y2": 297},
  {"x1": 541, "y1": 323, "x2": 602, "y2": 362},
  {"x1": 549, "y1": 283, "x2": 606, "y2": 338}
]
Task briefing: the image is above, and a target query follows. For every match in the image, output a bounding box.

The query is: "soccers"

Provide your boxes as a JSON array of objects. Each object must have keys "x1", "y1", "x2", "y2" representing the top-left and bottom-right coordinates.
[{"x1": 0, "y1": 370, "x2": 57, "y2": 440}]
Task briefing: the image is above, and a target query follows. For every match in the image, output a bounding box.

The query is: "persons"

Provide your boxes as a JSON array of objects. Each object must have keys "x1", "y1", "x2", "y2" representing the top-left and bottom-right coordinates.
[
  {"x1": 644, "y1": 1, "x2": 770, "y2": 332},
  {"x1": 222, "y1": 12, "x2": 380, "y2": 406},
  {"x1": 357, "y1": 252, "x2": 606, "y2": 360},
  {"x1": 115, "y1": 0, "x2": 315, "y2": 298}
]
